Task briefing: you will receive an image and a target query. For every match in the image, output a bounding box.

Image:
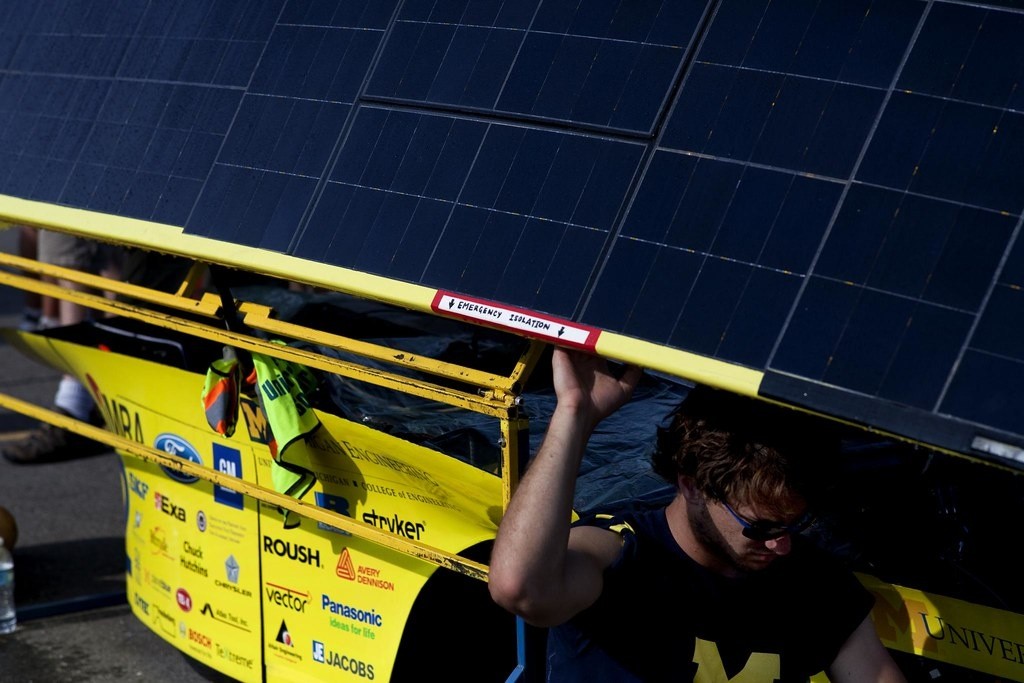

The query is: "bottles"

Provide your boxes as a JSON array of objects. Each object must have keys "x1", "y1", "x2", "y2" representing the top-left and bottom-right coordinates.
[{"x1": 0, "y1": 538, "x2": 17, "y2": 634}]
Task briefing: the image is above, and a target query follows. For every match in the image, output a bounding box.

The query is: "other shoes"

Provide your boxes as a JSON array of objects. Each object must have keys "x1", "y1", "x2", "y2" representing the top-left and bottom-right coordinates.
[
  {"x1": 0, "y1": 405, "x2": 113, "y2": 465},
  {"x1": 18, "y1": 312, "x2": 56, "y2": 331}
]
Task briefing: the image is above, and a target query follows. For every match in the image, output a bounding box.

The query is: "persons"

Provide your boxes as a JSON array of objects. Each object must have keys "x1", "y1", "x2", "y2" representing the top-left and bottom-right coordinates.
[
  {"x1": 16, "y1": 225, "x2": 61, "y2": 329},
  {"x1": 2, "y1": 229, "x2": 126, "y2": 463},
  {"x1": 488, "y1": 346, "x2": 907, "y2": 683}
]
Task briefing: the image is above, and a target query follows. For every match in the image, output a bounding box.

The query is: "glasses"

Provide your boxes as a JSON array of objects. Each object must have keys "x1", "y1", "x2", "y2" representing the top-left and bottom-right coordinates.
[{"x1": 707, "y1": 494, "x2": 817, "y2": 543}]
